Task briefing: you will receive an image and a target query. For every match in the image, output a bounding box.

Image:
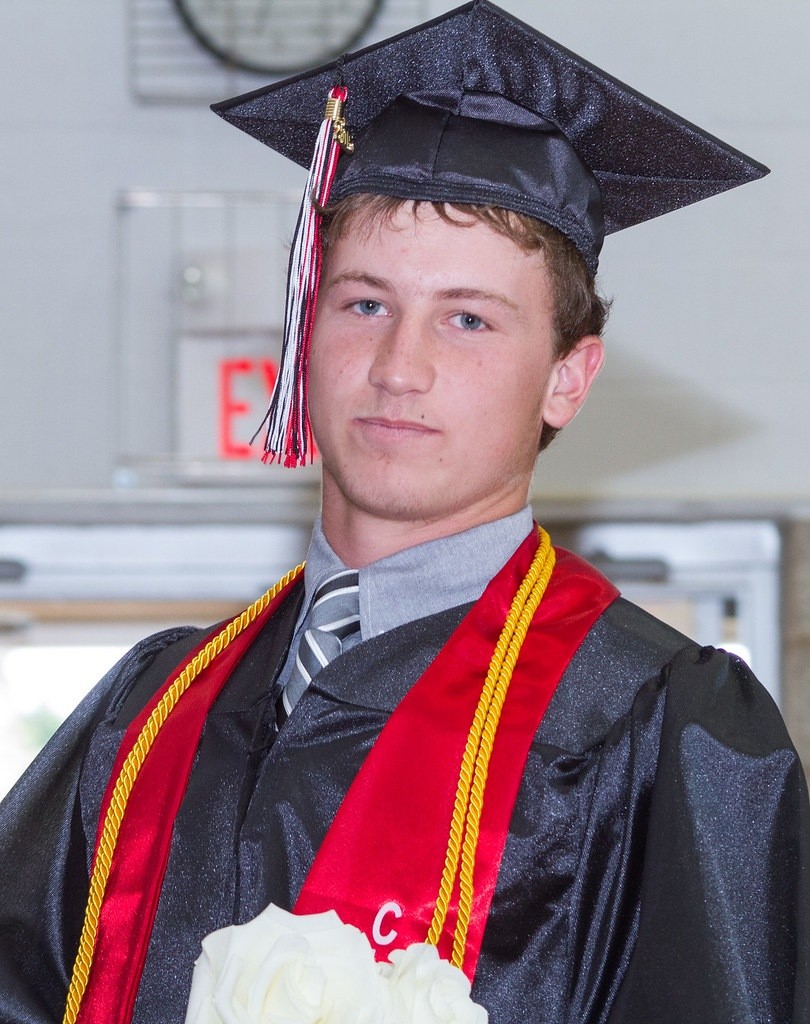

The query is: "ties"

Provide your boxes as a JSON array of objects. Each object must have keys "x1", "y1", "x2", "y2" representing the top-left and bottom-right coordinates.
[{"x1": 280, "y1": 569, "x2": 361, "y2": 717}]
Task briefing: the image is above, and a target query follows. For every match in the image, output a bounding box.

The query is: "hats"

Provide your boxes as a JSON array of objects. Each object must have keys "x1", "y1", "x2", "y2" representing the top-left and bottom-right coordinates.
[{"x1": 208, "y1": 0, "x2": 769, "y2": 482}]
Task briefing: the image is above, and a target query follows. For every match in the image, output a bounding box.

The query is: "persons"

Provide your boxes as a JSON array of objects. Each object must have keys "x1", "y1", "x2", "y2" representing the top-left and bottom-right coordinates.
[{"x1": 0, "y1": 0, "x2": 810, "y2": 1024}]
